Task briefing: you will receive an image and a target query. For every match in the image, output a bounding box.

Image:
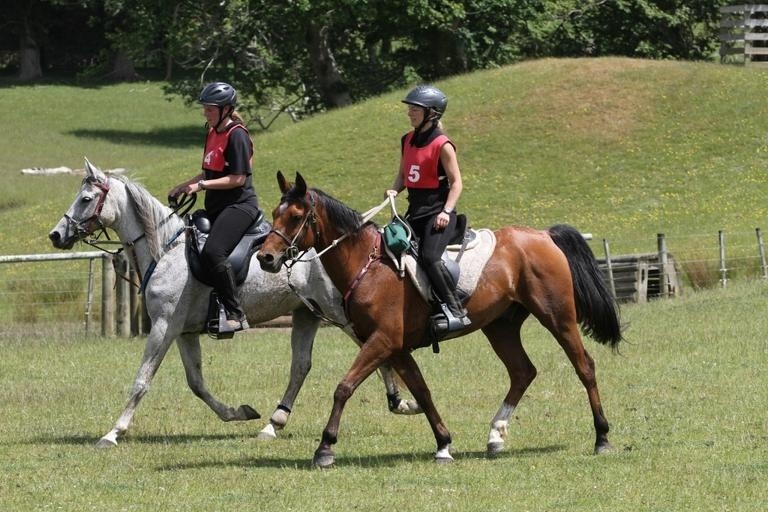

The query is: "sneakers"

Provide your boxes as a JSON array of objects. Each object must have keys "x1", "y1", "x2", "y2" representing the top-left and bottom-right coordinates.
[
  {"x1": 429, "y1": 315, "x2": 473, "y2": 337},
  {"x1": 208, "y1": 305, "x2": 251, "y2": 333}
]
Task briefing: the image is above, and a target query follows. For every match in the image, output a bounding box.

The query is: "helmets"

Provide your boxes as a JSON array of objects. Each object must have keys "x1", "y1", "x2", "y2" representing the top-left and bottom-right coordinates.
[
  {"x1": 401, "y1": 85, "x2": 448, "y2": 120},
  {"x1": 196, "y1": 82, "x2": 238, "y2": 107}
]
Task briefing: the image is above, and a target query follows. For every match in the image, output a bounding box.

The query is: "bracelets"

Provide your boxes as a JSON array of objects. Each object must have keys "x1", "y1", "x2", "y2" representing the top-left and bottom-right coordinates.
[{"x1": 197, "y1": 178, "x2": 206, "y2": 192}]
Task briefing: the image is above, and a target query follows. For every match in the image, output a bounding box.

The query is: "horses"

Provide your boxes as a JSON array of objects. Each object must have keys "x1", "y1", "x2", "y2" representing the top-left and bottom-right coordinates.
[
  {"x1": 257, "y1": 171, "x2": 637, "y2": 468},
  {"x1": 48, "y1": 157, "x2": 423, "y2": 450}
]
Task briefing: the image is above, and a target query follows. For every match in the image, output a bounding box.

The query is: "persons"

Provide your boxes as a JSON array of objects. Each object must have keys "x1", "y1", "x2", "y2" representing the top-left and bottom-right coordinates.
[
  {"x1": 168, "y1": 80, "x2": 260, "y2": 332},
  {"x1": 386, "y1": 85, "x2": 472, "y2": 332}
]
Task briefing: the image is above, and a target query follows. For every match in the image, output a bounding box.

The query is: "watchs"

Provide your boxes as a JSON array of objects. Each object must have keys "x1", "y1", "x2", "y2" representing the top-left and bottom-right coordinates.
[{"x1": 442, "y1": 208, "x2": 453, "y2": 217}]
